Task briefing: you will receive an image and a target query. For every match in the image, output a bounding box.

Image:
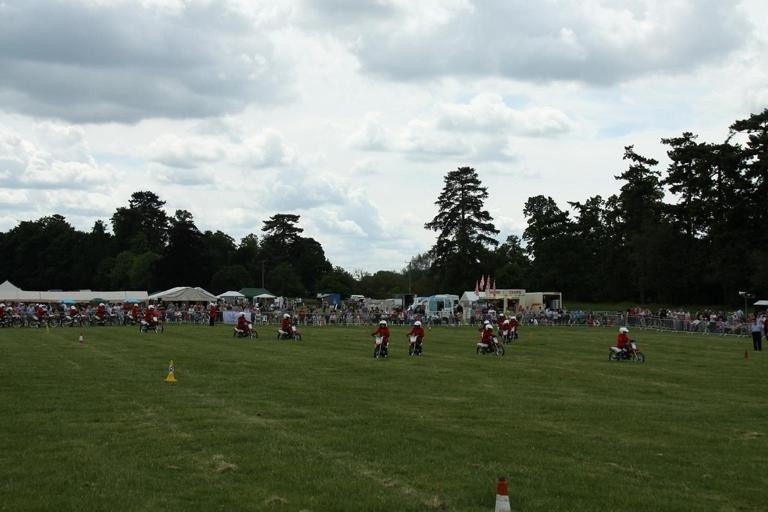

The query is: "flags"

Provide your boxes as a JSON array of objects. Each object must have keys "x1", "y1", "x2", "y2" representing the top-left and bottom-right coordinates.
[{"x1": 475, "y1": 275, "x2": 495, "y2": 296}]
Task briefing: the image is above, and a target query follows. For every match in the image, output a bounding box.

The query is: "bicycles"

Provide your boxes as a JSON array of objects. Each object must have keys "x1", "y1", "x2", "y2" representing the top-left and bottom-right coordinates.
[
  {"x1": 277, "y1": 321, "x2": 302, "y2": 341},
  {"x1": 234, "y1": 322, "x2": 258, "y2": 339}
]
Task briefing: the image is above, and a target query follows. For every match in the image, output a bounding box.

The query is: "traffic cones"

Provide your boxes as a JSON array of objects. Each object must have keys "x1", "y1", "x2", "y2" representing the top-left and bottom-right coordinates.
[
  {"x1": 79, "y1": 332, "x2": 84, "y2": 345},
  {"x1": 744, "y1": 350, "x2": 749, "y2": 360},
  {"x1": 165, "y1": 359, "x2": 178, "y2": 382},
  {"x1": 493, "y1": 476, "x2": 512, "y2": 512}
]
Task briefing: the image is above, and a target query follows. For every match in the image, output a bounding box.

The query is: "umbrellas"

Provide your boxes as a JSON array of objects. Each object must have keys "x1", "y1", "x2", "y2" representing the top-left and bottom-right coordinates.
[{"x1": 148, "y1": 287, "x2": 276, "y2": 301}]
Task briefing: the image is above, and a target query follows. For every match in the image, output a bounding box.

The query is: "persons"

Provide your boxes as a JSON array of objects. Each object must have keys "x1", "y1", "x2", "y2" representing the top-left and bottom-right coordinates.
[
  {"x1": 626, "y1": 306, "x2": 768, "y2": 351},
  {"x1": 425, "y1": 306, "x2": 623, "y2": 328},
  {"x1": 282, "y1": 313, "x2": 293, "y2": 339},
  {"x1": 617, "y1": 327, "x2": 631, "y2": 359},
  {"x1": 297, "y1": 305, "x2": 425, "y2": 328},
  {"x1": 407, "y1": 322, "x2": 424, "y2": 354},
  {"x1": 498, "y1": 314, "x2": 518, "y2": 343},
  {"x1": 481, "y1": 320, "x2": 497, "y2": 351},
  {"x1": 372, "y1": 320, "x2": 389, "y2": 354},
  {"x1": 0, "y1": 300, "x2": 273, "y2": 325},
  {"x1": 237, "y1": 314, "x2": 252, "y2": 337},
  {"x1": 143, "y1": 305, "x2": 161, "y2": 330}
]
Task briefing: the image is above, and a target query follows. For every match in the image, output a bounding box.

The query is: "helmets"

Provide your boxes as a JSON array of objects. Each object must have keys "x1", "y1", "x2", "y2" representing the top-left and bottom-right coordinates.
[
  {"x1": 414, "y1": 321, "x2": 421, "y2": 328},
  {"x1": 284, "y1": 314, "x2": 290, "y2": 318},
  {"x1": 239, "y1": 312, "x2": 245, "y2": 318},
  {"x1": 484, "y1": 313, "x2": 516, "y2": 331},
  {"x1": 619, "y1": 327, "x2": 628, "y2": 334},
  {"x1": 379, "y1": 320, "x2": 386, "y2": 327},
  {"x1": 0, "y1": 302, "x2": 155, "y2": 311}
]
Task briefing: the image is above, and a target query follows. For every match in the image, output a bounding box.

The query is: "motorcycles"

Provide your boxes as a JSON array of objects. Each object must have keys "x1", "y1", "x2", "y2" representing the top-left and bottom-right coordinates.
[
  {"x1": 608, "y1": 339, "x2": 644, "y2": 365},
  {"x1": 0, "y1": 314, "x2": 164, "y2": 335},
  {"x1": 475, "y1": 313, "x2": 520, "y2": 357},
  {"x1": 372, "y1": 334, "x2": 423, "y2": 358}
]
